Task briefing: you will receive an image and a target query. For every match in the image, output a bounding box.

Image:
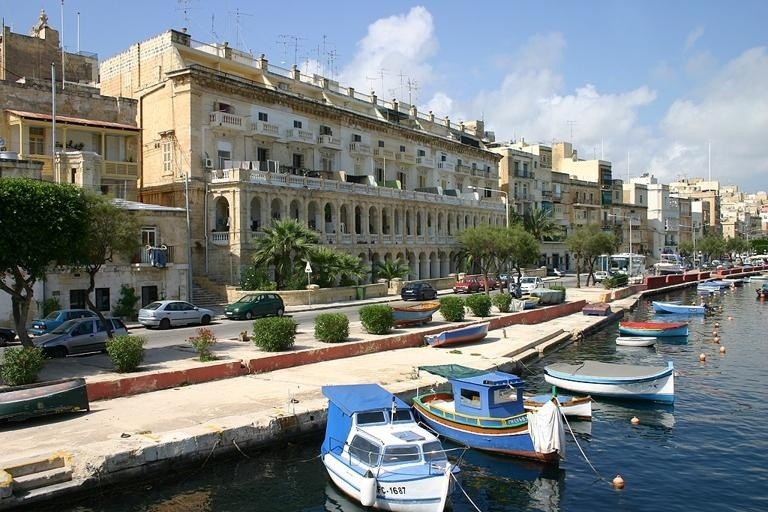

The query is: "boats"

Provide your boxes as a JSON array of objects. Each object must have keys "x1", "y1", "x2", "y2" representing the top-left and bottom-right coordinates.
[
  {"x1": 412, "y1": 363, "x2": 562, "y2": 461},
  {"x1": 617, "y1": 320, "x2": 688, "y2": 337},
  {"x1": 389, "y1": 300, "x2": 441, "y2": 327},
  {"x1": 542, "y1": 356, "x2": 674, "y2": 405},
  {"x1": 321, "y1": 479, "x2": 363, "y2": 512},
  {"x1": 548, "y1": 387, "x2": 677, "y2": 434},
  {"x1": 424, "y1": 322, "x2": 490, "y2": 347},
  {"x1": 652, "y1": 300, "x2": 706, "y2": 314},
  {"x1": 649, "y1": 314, "x2": 705, "y2": 325},
  {"x1": 317, "y1": 381, "x2": 471, "y2": 512},
  {"x1": 512, "y1": 295, "x2": 540, "y2": 311},
  {"x1": 695, "y1": 272, "x2": 768, "y2": 291},
  {"x1": 615, "y1": 336, "x2": 658, "y2": 347},
  {"x1": 508, "y1": 387, "x2": 592, "y2": 423},
  {"x1": 755, "y1": 283, "x2": 768, "y2": 298},
  {"x1": 436, "y1": 436, "x2": 565, "y2": 511},
  {"x1": 583, "y1": 301, "x2": 611, "y2": 317},
  {"x1": 655, "y1": 333, "x2": 689, "y2": 352}
]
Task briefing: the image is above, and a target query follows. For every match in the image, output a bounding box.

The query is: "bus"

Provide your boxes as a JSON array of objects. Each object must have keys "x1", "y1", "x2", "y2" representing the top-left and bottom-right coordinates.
[{"x1": 610, "y1": 252, "x2": 646, "y2": 278}]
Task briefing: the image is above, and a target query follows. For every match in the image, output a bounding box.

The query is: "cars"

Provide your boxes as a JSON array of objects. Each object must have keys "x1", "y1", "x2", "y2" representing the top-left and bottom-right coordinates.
[
  {"x1": 223, "y1": 292, "x2": 286, "y2": 319},
  {"x1": 653, "y1": 251, "x2": 768, "y2": 273},
  {"x1": 400, "y1": 281, "x2": 438, "y2": 301},
  {"x1": 451, "y1": 272, "x2": 527, "y2": 300},
  {"x1": 592, "y1": 270, "x2": 610, "y2": 284},
  {"x1": 520, "y1": 277, "x2": 545, "y2": 294},
  {"x1": 0, "y1": 326, "x2": 18, "y2": 346},
  {"x1": 31, "y1": 315, "x2": 128, "y2": 358},
  {"x1": 137, "y1": 300, "x2": 215, "y2": 330},
  {"x1": 30, "y1": 307, "x2": 100, "y2": 335},
  {"x1": 546, "y1": 268, "x2": 565, "y2": 278}
]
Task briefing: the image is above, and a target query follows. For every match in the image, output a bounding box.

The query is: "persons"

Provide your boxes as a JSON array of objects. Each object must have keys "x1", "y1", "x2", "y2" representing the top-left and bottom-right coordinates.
[
  {"x1": 517, "y1": 283, "x2": 523, "y2": 298},
  {"x1": 509, "y1": 282, "x2": 518, "y2": 295},
  {"x1": 618, "y1": 266, "x2": 625, "y2": 275}
]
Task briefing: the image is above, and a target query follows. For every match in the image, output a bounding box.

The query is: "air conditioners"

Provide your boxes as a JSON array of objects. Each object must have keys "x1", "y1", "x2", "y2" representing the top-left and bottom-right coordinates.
[{"x1": 204, "y1": 158, "x2": 214, "y2": 168}]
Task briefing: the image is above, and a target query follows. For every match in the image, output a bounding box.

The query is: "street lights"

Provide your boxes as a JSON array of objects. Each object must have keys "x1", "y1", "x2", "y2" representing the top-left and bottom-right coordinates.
[
  {"x1": 466, "y1": 186, "x2": 509, "y2": 239},
  {"x1": 607, "y1": 212, "x2": 633, "y2": 278},
  {"x1": 677, "y1": 223, "x2": 709, "y2": 269}
]
